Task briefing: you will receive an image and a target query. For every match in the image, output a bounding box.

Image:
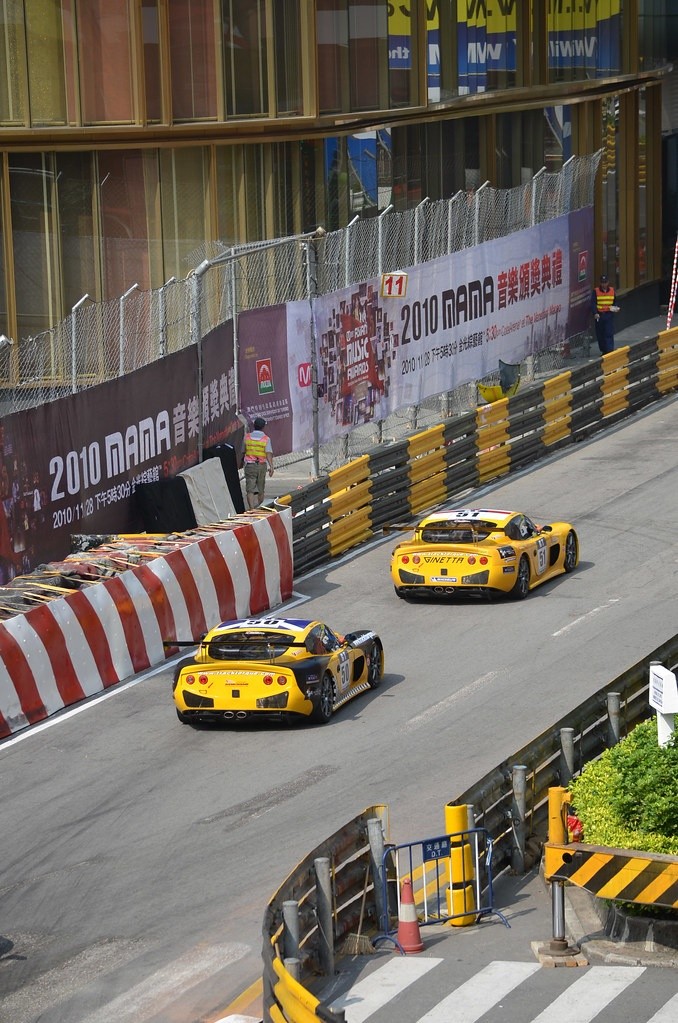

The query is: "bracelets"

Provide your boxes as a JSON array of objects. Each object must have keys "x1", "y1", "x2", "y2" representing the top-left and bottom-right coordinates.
[{"x1": 269, "y1": 464, "x2": 273, "y2": 466}]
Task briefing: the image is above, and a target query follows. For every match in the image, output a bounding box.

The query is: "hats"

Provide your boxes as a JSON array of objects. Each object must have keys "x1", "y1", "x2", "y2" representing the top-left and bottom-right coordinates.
[
  {"x1": 600, "y1": 274, "x2": 609, "y2": 282},
  {"x1": 255, "y1": 418, "x2": 265, "y2": 427}
]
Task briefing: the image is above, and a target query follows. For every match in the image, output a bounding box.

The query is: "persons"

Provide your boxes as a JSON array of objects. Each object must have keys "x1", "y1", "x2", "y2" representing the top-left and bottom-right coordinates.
[
  {"x1": 317, "y1": 284, "x2": 398, "y2": 426},
  {"x1": 590, "y1": 275, "x2": 620, "y2": 357},
  {"x1": 241, "y1": 418, "x2": 274, "y2": 509}
]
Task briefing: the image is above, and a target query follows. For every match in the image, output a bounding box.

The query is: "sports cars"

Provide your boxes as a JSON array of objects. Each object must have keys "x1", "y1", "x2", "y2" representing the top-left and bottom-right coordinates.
[
  {"x1": 161, "y1": 617, "x2": 384, "y2": 729},
  {"x1": 382, "y1": 508, "x2": 581, "y2": 604}
]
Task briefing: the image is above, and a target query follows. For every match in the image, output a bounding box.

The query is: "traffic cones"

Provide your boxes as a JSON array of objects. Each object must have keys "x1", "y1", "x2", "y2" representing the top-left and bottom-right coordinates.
[{"x1": 394, "y1": 877, "x2": 426, "y2": 954}]
